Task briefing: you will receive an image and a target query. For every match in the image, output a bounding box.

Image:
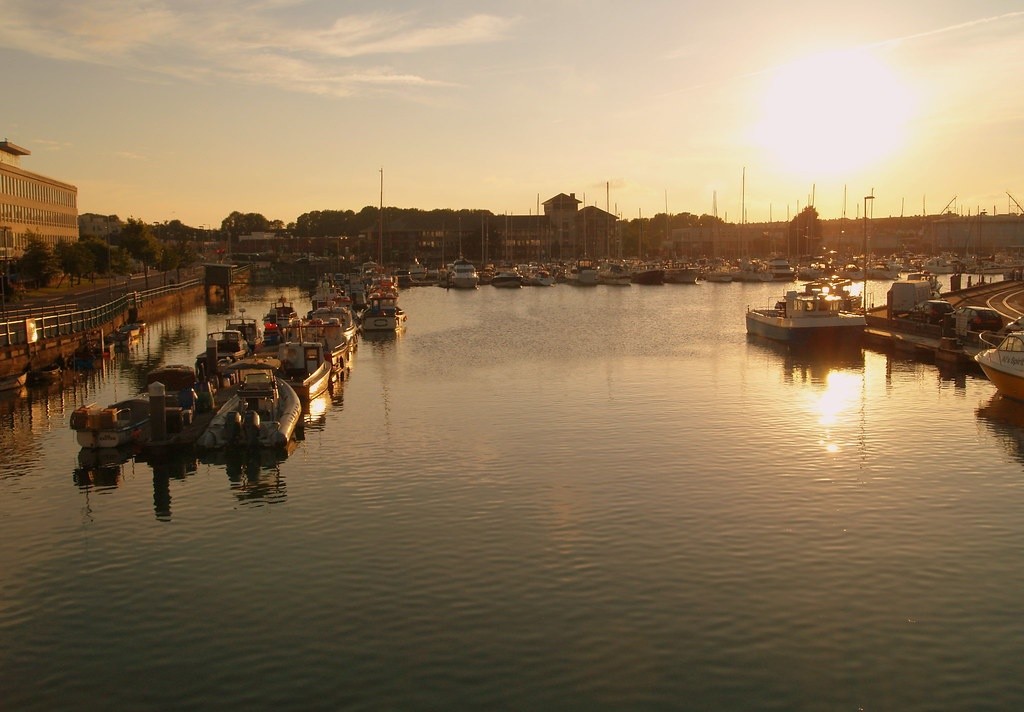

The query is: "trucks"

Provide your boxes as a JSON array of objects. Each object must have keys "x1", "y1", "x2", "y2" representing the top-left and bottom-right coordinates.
[{"x1": 891, "y1": 280, "x2": 945, "y2": 318}]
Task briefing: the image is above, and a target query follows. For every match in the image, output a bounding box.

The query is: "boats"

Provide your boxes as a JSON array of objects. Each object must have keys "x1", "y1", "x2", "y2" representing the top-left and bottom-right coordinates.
[
  {"x1": 744, "y1": 275, "x2": 868, "y2": 347},
  {"x1": 0, "y1": 371, "x2": 28, "y2": 391},
  {"x1": 67, "y1": 258, "x2": 407, "y2": 464},
  {"x1": 30, "y1": 361, "x2": 62, "y2": 382},
  {"x1": 975, "y1": 330, "x2": 1023, "y2": 403}
]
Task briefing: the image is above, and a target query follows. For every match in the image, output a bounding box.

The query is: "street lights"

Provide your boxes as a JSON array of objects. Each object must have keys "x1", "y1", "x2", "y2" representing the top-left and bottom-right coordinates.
[
  {"x1": 979, "y1": 211, "x2": 988, "y2": 287},
  {"x1": 864, "y1": 195, "x2": 875, "y2": 315}
]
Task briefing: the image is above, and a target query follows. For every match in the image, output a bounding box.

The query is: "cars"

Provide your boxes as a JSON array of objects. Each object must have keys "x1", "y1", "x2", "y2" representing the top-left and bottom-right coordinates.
[
  {"x1": 1004, "y1": 315, "x2": 1024, "y2": 336},
  {"x1": 907, "y1": 300, "x2": 956, "y2": 326},
  {"x1": 943, "y1": 306, "x2": 1003, "y2": 337}
]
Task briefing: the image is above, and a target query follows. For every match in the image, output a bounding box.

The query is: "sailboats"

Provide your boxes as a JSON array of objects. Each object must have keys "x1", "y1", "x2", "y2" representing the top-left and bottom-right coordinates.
[{"x1": 411, "y1": 166, "x2": 1024, "y2": 288}]
